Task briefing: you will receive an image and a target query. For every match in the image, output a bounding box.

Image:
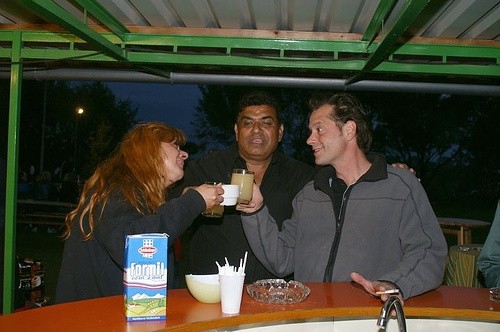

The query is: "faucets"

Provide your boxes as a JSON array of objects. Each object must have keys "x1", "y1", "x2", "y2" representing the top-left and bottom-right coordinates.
[{"x1": 377, "y1": 295, "x2": 406, "y2": 332}]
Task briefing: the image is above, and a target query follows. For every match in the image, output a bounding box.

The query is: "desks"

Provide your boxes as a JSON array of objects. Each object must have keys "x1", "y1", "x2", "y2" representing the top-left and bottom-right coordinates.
[
  {"x1": 443, "y1": 244, "x2": 486, "y2": 287},
  {"x1": 436, "y1": 217, "x2": 491, "y2": 246}
]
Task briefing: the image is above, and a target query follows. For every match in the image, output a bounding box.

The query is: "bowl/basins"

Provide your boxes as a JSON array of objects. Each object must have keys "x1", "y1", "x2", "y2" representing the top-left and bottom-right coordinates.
[{"x1": 185, "y1": 273, "x2": 224, "y2": 304}]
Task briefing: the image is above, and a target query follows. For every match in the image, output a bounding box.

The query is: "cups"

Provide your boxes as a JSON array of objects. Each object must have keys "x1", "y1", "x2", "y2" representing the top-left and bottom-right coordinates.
[
  {"x1": 201, "y1": 182, "x2": 224, "y2": 218},
  {"x1": 219, "y1": 184, "x2": 240, "y2": 206},
  {"x1": 230, "y1": 168, "x2": 255, "y2": 204},
  {"x1": 218, "y1": 271, "x2": 246, "y2": 315}
]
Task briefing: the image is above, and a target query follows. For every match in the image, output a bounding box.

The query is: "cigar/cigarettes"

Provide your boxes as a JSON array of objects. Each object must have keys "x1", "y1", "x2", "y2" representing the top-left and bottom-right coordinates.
[{"x1": 376, "y1": 290, "x2": 399, "y2": 294}]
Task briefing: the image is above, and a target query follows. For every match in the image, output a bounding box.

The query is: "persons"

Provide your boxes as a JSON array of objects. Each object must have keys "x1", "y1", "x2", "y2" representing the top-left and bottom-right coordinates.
[
  {"x1": 476, "y1": 199, "x2": 500, "y2": 290},
  {"x1": 236, "y1": 89, "x2": 448, "y2": 305},
  {"x1": 167, "y1": 92, "x2": 408, "y2": 289},
  {"x1": 29, "y1": 171, "x2": 55, "y2": 231},
  {"x1": 59, "y1": 172, "x2": 78, "y2": 213},
  {"x1": 55, "y1": 121, "x2": 224, "y2": 304}
]
